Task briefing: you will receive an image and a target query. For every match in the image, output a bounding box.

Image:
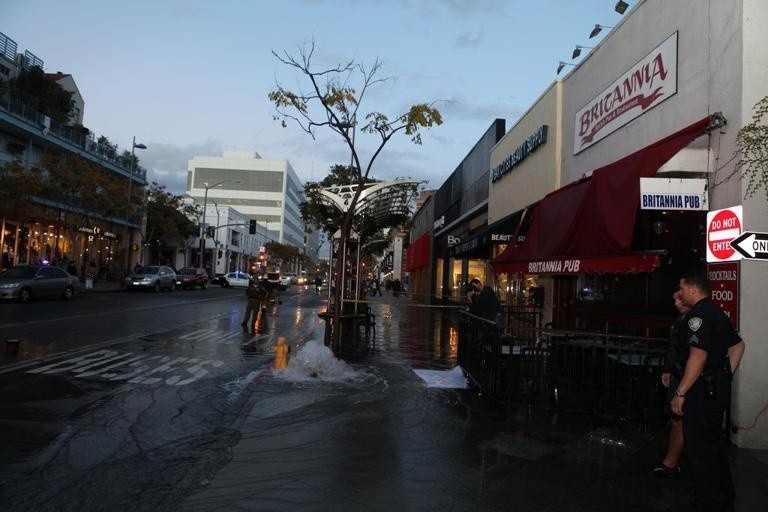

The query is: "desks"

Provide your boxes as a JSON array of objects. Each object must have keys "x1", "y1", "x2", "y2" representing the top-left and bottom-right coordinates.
[
  {"x1": 541, "y1": 330, "x2": 681, "y2": 418},
  {"x1": 482, "y1": 343, "x2": 551, "y2": 415}
]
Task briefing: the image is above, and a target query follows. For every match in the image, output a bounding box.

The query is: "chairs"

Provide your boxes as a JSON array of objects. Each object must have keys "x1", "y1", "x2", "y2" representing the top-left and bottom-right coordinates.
[{"x1": 520, "y1": 346, "x2": 559, "y2": 419}]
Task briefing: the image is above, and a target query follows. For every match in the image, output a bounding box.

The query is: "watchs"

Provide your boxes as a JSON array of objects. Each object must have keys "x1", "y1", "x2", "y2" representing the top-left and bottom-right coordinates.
[{"x1": 673, "y1": 390, "x2": 686, "y2": 398}]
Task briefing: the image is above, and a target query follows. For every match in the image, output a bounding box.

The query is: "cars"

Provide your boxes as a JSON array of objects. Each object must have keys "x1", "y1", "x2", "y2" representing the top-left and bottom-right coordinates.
[
  {"x1": 125, "y1": 265, "x2": 307, "y2": 293},
  {"x1": 0, "y1": 264, "x2": 82, "y2": 303}
]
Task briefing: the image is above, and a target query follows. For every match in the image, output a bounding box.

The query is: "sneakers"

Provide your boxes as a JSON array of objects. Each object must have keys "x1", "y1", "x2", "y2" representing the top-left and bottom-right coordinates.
[{"x1": 648, "y1": 461, "x2": 672, "y2": 480}]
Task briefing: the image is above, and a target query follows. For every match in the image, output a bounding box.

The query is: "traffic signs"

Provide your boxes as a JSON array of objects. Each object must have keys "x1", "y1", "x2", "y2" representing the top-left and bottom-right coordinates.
[{"x1": 730, "y1": 230, "x2": 768, "y2": 261}]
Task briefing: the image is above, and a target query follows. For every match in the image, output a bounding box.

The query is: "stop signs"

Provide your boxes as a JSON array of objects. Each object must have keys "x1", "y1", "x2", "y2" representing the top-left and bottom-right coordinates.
[{"x1": 706, "y1": 205, "x2": 743, "y2": 263}]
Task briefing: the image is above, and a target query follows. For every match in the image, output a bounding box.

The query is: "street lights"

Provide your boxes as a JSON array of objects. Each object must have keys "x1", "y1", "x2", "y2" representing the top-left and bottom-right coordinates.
[
  {"x1": 127, "y1": 136, "x2": 146, "y2": 272},
  {"x1": 199, "y1": 179, "x2": 241, "y2": 267}
]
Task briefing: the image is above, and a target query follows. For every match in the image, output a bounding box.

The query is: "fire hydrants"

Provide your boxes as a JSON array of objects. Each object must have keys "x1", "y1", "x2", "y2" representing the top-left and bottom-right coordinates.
[{"x1": 272, "y1": 336, "x2": 291, "y2": 368}]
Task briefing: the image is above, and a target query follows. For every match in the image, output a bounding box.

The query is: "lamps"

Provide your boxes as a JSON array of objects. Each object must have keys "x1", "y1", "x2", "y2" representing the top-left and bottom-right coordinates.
[{"x1": 557, "y1": 0, "x2": 629, "y2": 75}]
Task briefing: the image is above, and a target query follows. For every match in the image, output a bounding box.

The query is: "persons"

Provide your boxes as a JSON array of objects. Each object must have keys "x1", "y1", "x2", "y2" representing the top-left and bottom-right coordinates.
[
  {"x1": 367, "y1": 274, "x2": 402, "y2": 298},
  {"x1": 669, "y1": 268, "x2": 746, "y2": 512},
  {"x1": 468, "y1": 278, "x2": 505, "y2": 354},
  {"x1": 67, "y1": 261, "x2": 79, "y2": 275},
  {"x1": 648, "y1": 287, "x2": 692, "y2": 474},
  {"x1": 132, "y1": 260, "x2": 144, "y2": 273},
  {"x1": 240, "y1": 277, "x2": 265, "y2": 327}
]
